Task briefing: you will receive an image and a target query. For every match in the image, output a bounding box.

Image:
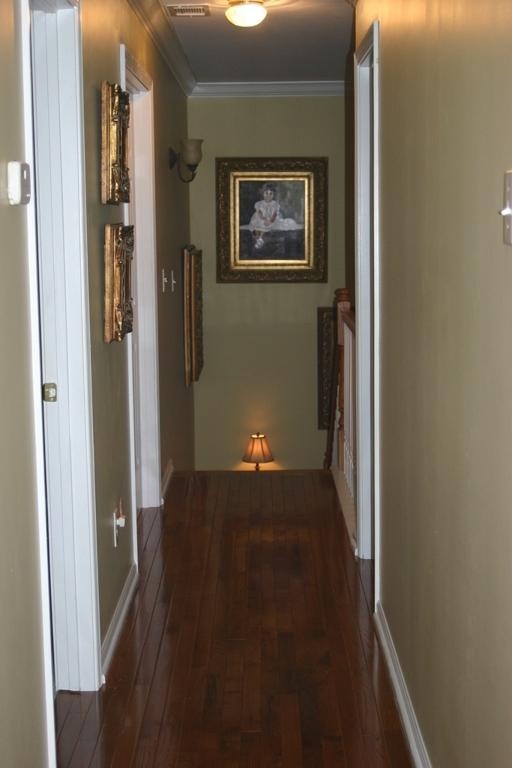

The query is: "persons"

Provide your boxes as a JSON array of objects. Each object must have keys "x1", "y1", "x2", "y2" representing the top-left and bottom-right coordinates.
[{"x1": 246, "y1": 180, "x2": 281, "y2": 249}]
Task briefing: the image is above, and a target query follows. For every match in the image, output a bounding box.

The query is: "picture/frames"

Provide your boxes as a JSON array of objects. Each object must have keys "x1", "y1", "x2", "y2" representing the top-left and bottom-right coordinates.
[
  {"x1": 183, "y1": 246, "x2": 203, "y2": 382},
  {"x1": 102, "y1": 224, "x2": 137, "y2": 339},
  {"x1": 102, "y1": 80, "x2": 132, "y2": 206},
  {"x1": 213, "y1": 155, "x2": 329, "y2": 283},
  {"x1": 316, "y1": 306, "x2": 333, "y2": 429}
]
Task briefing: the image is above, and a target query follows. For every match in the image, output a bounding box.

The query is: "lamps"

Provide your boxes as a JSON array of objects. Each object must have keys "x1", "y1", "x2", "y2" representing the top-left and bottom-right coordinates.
[
  {"x1": 240, "y1": 430, "x2": 274, "y2": 472},
  {"x1": 166, "y1": 136, "x2": 206, "y2": 181},
  {"x1": 221, "y1": 0, "x2": 268, "y2": 28}
]
